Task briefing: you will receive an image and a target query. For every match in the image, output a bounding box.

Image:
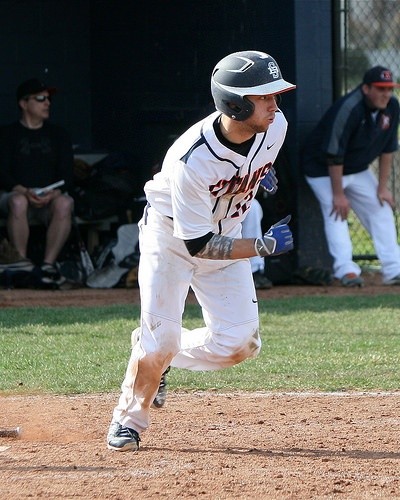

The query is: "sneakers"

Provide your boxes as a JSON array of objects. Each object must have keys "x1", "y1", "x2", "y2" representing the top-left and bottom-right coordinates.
[
  {"x1": 131, "y1": 326, "x2": 171, "y2": 411},
  {"x1": 342, "y1": 273, "x2": 363, "y2": 288},
  {"x1": 386, "y1": 275, "x2": 400, "y2": 285},
  {"x1": 107, "y1": 418, "x2": 140, "y2": 453}
]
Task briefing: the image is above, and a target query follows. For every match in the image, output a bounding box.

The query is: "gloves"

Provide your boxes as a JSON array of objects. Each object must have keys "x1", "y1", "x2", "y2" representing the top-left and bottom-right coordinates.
[
  {"x1": 260, "y1": 165, "x2": 279, "y2": 195},
  {"x1": 255, "y1": 215, "x2": 294, "y2": 257}
]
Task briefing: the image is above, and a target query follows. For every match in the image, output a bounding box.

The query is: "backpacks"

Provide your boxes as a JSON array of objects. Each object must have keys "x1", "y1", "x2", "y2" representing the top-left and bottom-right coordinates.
[{"x1": 72, "y1": 153, "x2": 140, "y2": 221}]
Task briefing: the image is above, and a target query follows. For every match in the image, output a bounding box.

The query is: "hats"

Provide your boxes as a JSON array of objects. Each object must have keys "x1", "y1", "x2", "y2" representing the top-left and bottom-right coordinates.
[
  {"x1": 363, "y1": 65, "x2": 400, "y2": 88},
  {"x1": 16, "y1": 78, "x2": 55, "y2": 100}
]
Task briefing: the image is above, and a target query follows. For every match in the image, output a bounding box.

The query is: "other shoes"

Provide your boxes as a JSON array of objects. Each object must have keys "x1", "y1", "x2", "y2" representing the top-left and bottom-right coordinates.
[{"x1": 40, "y1": 264, "x2": 62, "y2": 285}]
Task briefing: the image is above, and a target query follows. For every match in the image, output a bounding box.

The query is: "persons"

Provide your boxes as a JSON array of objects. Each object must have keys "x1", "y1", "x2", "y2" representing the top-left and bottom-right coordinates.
[
  {"x1": 107, "y1": 51, "x2": 297, "y2": 451},
  {"x1": 303, "y1": 66, "x2": 400, "y2": 287},
  {"x1": 0, "y1": 79, "x2": 80, "y2": 284}
]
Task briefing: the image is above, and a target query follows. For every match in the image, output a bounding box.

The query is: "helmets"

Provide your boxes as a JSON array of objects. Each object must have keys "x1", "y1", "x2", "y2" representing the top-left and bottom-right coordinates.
[{"x1": 209, "y1": 51, "x2": 296, "y2": 121}]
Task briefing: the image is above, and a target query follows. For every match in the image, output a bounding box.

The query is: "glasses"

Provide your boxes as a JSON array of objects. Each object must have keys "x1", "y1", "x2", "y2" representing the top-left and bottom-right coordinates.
[{"x1": 26, "y1": 95, "x2": 52, "y2": 102}]
